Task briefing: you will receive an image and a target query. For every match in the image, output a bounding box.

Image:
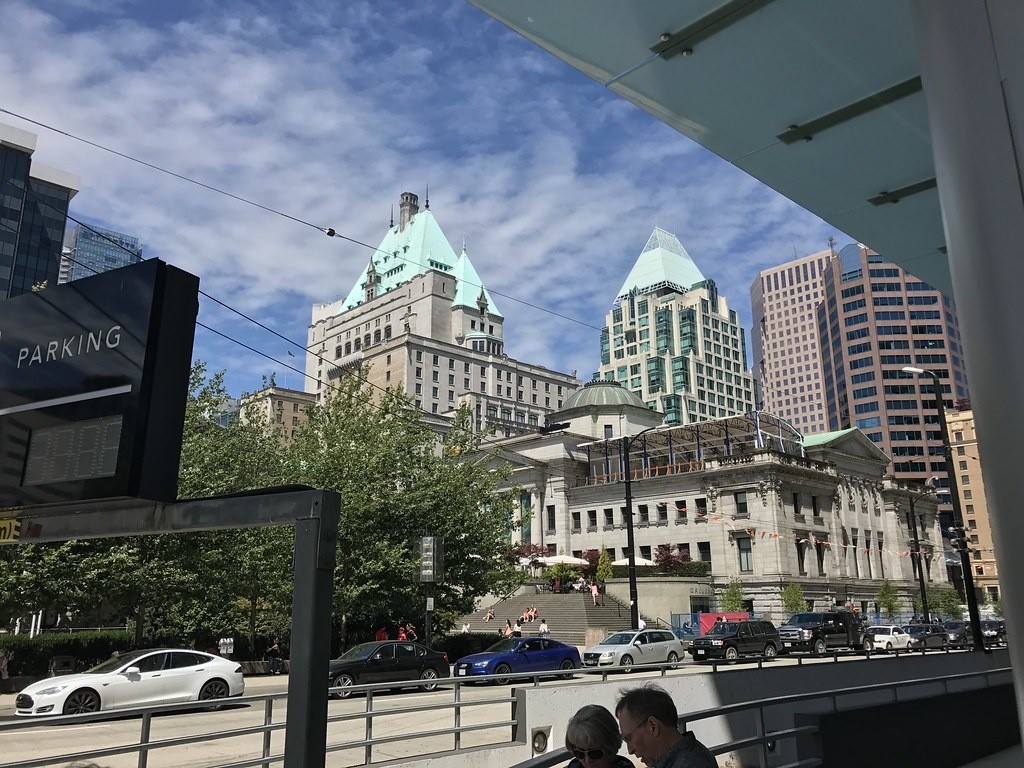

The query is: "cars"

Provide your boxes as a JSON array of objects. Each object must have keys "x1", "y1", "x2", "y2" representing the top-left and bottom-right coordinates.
[
  {"x1": 867, "y1": 626, "x2": 913, "y2": 655},
  {"x1": 454, "y1": 637, "x2": 582, "y2": 686},
  {"x1": 979, "y1": 619, "x2": 1008, "y2": 647},
  {"x1": 942, "y1": 621, "x2": 974, "y2": 650},
  {"x1": 14, "y1": 647, "x2": 245, "y2": 724},
  {"x1": 902, "y1": 624, "x2": 950, "y2": 651},
  {"x1": 328, "y1": 640, "x2": 450, "y2": 700}
]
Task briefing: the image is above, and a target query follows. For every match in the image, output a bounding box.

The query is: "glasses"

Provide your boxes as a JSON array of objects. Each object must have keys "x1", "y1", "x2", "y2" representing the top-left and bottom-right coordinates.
[
  {"x1": 568, "y1": 745, "x2": 607, "y2": 760},
  {"x1": 622, "y1": 718, "x2": 649, "y2": 745}
]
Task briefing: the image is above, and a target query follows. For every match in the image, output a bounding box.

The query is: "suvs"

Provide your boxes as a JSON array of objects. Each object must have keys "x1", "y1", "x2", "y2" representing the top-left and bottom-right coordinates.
[
  {"x1": 581, "y1": 627, "x2": 686, "y2": 674},
  {"x1": 776, "y1": 611, "x2": 875, "y2": 658},
  {"x1": 688, "y1": 618, "x2": 783, "y2": 666}
]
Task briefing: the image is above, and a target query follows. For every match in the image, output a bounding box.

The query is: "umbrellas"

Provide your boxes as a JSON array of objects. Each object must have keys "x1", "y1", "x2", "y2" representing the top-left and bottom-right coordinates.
[
  {"x1": 611, "y1": 556, "x2": 659, "y2": 566},
  {"x1": 532, "y1": 554, "x2": 590, "y2": 573}
]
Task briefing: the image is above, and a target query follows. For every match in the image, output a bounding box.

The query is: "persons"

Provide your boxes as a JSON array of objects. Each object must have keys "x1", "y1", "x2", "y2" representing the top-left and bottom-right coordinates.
[
  {"x1": 376, "y1": 625, "x2": 388, "y2": 641},
  {"x1": 266, "y1": 638, "x2": 281, "y2": 674},
  {"x1": 910, "y1": 615, "x2": 943, "y2": 626},
  {"x1": 713, "y1": 616, "x2": 729, "y2": 632},
  {"x1": 538, "y1": 619, "x2": 551, "y2": 638},
  {"x1": 696, "y1": 608, "x2": 702, "y2": 612},
  {"x1": 398, "y1": 623, "x2": 417, "y2": 641},
  {"x1": 562, "y1": 681, "x2": 719, "y2": 768},
  {"x1": 498, "y1": 627, "x2": 502, "y2": 634},
  {"x1": 461, "y1": 620, "x2": 470, "y2": 633},
  {"x1": 186, "y1": 638, "x2": 195, "y2": 651},
  {"x1": 548, "y1": 575, "x2": 579, "y2": 594},
  {"x1": 513, "y1": 619, "x2": 521, "y2": 637},
  {"x1": 590, "y1": 582, "x2": 598, "y2": 607},
  {"x1": 519, "y1": 604, "x2": 538, "y2": 624},
  {"x1": 504, "y1": 619, "x2": 513, "y2": 636},
  {"x1": 483, "y1": 605, "x2": 494, "y2": 622},
  {"x1": 683, "y1": 621, "x2": 694, "y2": 634}
]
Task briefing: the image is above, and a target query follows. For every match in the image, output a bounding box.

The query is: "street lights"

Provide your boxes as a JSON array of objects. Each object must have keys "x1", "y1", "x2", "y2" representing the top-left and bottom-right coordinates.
[
  {"x1": 909, "y1": 490, "x2": 949, "y2": 624},
  {"x1": 623, "y1": 424, "x2": 670, "y2": 630},
  {"x1": 902, "y1": 366, "x2": 982, "y2": 648}
]
